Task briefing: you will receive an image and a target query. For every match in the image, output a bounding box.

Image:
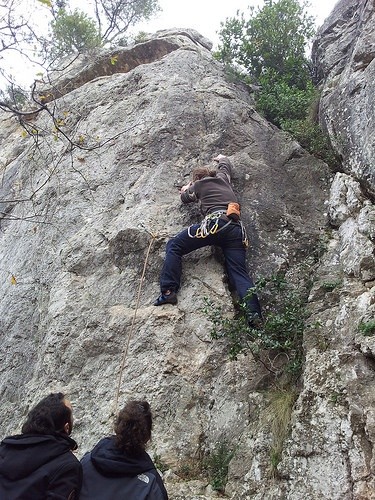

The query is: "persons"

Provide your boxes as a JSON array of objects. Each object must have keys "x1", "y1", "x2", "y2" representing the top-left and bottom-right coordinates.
[
  {"x1": 153, "y1": 155, "x2": 263, "y2": 331},
  {"x1": 0, "y1": 392, "x2": 83, "y2": 500},
  {"x1": 80, "y1": 401, "x2": 168, "y2": 500}
]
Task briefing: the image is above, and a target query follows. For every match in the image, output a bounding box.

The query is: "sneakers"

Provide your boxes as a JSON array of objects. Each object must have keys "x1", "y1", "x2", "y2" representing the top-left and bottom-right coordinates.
[
  {"x1": 248, "y1": 319, "x2": 263, "y2": 330},
  {"x1": 154, "y1": 291, "x2": 177, "y2": 306}
]
